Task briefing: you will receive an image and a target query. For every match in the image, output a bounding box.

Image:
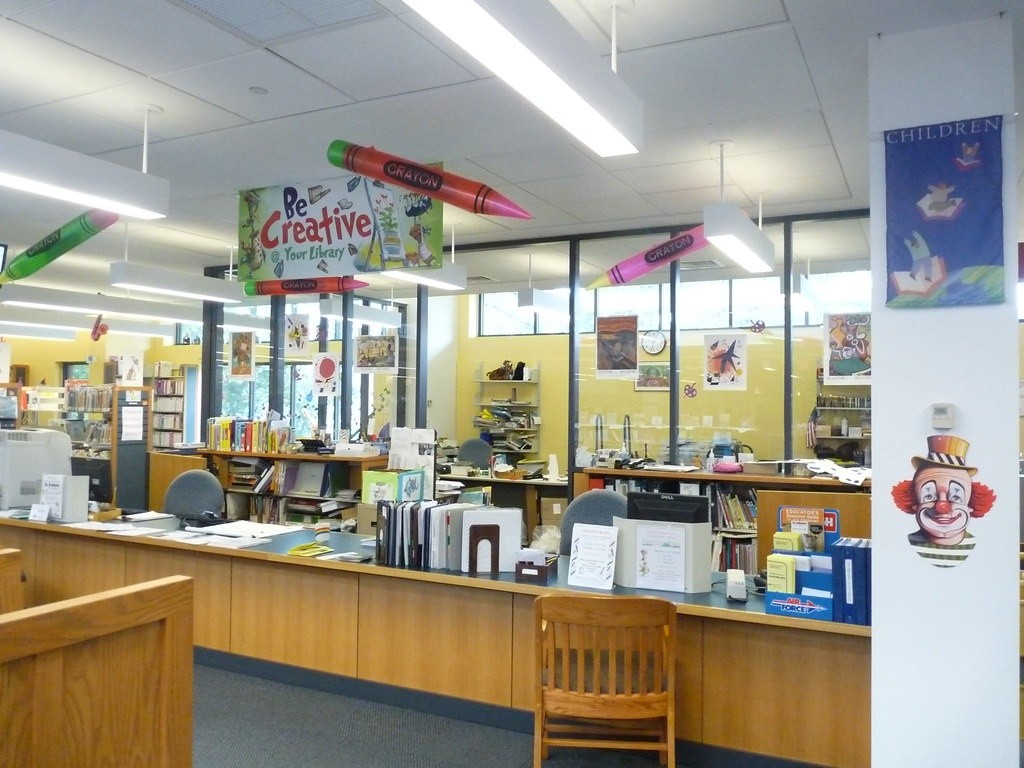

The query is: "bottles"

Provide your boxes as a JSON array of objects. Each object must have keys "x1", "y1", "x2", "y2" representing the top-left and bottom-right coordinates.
[
  {"x1": 706, "y1": 449, "x2": 715, "y2": 471},
  {"x1": 864, "y1": 446, "x2": 871, "y2": 467},
  {"x1": 841, "y1": 418, "x2": 848, "y2": 436}
]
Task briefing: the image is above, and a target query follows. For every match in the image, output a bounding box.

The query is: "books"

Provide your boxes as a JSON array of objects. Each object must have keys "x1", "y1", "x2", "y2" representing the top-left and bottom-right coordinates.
[
  {"x1": 706, "y1": 483, "x2": 760, "y2": 575},
  {"x1": 151, "y1": 362, "x2": 186, "y2": 449},
  {"x1": 249, "y1": 497, "x2": 281, "y2": 521},
  {"x1": 376, "y1": 499, "x2": 524, "y2": 574},
  {"x1": 206, "y1": 409, "x2": 297, "y2": 454},
  {"x1": 64, "y1": 385, "x2": 117, "y2": 446}
]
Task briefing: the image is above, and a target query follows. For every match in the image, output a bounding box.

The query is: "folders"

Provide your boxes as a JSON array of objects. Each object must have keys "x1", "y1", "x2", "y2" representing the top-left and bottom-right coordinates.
[{"x1": 831, "y1": 537, "x2": 872, "y2": 627}]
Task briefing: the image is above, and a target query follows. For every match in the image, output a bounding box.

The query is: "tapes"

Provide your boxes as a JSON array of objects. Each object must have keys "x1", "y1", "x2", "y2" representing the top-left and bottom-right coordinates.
[{"x1": 90, "y1": 508, "x2": 122, "y2": 522}]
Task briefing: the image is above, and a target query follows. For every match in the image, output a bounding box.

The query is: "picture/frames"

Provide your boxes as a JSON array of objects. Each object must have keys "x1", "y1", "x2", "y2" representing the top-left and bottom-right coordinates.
[{"x1": 635, "y1": 360, "x2": 671, "y2": 391}]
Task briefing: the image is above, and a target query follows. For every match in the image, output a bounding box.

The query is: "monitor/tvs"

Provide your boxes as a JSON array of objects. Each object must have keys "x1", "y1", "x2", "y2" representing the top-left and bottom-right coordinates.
[
  {"x1": 627, "y1": 492, "x2": 708, "y2": 523},
  {"x1": 71, "y1": 457, "x2": 113, "y2": 503}
]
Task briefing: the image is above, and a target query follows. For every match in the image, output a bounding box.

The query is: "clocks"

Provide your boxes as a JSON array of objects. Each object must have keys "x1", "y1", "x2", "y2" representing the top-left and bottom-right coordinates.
[{"x1": 642, "y1": 330, "x2": 665, "y2": 355}]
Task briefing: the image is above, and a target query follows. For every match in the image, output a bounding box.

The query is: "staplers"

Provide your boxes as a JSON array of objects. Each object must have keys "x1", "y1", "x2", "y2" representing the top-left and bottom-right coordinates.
[
  {"x1": 318, "y1": 448, "x2": 334, "y2": 454},
  {"x1": 532, "y1": 468, "x2": 543, "y2": 478},
  {"x1": 628, "y1": 460, "x2": 645, "y2": 469}
]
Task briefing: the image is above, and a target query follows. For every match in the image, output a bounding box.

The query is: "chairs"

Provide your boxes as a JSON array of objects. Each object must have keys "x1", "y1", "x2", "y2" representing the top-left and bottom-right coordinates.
[
  {"x1": 532, "y1": 592, "x2": 677, "y2": 768},
  {"x1": 458, "y1": 438, "x2": 493, "y2": 471},
  {"x1": 122, "y1": 469, "x2": 225, "y2": 528},
  {"x1": 560, "y1": 488, "x2": 628, "y2": 555}
]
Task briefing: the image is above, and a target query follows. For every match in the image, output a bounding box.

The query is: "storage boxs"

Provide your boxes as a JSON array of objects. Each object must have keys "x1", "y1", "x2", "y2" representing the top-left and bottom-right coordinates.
[
  {"x1": 541, "y1": 496, "x2": 568, "y2": 530},
  {"x1": 361, "y1": 466, "x2": 424, "y2": 503},
  {"x1": 678, "y1": 481, "x2": 700, "y2": 496},
  {"x1": 792, "y1": 463, "x2": 816, "y2": 478},
  {"x1": 741, "y1": 461, "x2": 779, "y2": 475},
  {"x1": 589, "y1": 478, "x2": 604, "y2": 491},
  {"x1": 357, "y1": 503, "x2": 377, "y2": 535}
]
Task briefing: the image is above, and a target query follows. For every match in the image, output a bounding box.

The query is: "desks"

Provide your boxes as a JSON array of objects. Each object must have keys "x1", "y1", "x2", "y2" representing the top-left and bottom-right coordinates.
[
  {"x1": 0, "y1": 507, "x2": 871, "y2": 767},
  {"x1": 196, "y1": 448, "x2": 387, "y2": 534}
]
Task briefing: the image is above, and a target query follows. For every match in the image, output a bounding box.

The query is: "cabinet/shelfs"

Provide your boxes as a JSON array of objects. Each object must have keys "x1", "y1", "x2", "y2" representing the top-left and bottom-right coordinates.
[
  {"x1": 144, "y1": 362, "x2": 198, "y2": 449},
  {"x1": 584, "y1": 466, "x2": 872, "y2": 574},
  {"x1": 21, "y1": 384, "x2": 153, "y2": 511},
  {"x1": 474, "y1": 360, "x2": 540, "y2": 455},
  {"x1": 816, "y1": 360, "x2": 872, "y2": 440}
]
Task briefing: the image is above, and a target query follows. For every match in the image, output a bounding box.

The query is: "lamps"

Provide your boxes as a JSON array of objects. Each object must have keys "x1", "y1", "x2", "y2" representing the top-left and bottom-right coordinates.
[
  {"x1": 319, "y1": 278, "x2": 402, "y2": 328},
  {"x1": 0, "y1": 222, "x2": 271, "y2": 343},
  {"x1": 701, "y1": 140, "x2": 776, "y2": 273},
  {"x1": 381, "y1": 225, "x2": 467, "y2": 292},
  {"x1": 0, "y1": 101, "x2": 171, "y2": 220},
  {"x1": 403, "y1": 0, "x2": 644, "y2": 158},
  {"x1": 518, "y1": 251, "x2": 563, "y2": 312}
]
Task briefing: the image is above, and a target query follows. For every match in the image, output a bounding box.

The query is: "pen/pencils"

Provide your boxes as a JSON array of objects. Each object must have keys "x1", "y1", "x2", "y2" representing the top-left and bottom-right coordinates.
[
  {"x1": 244, "y1": 276, "x2": 369, "y2": 295},
  {"x1": 0, "y1": 208, "x2": 119, "y2": 283},
  {"x1": 327, "y1": 139, "x2": 533, "y2": 220},
  {"x1": 645, "y1": 443, "x2": 647, "y2": 458},
  {"x1": 585, "y1": 225, "x2": 709, "y2": 290},
  {"x1": 546, "y1": 555, "x2": 560, "y2": 562}
]
